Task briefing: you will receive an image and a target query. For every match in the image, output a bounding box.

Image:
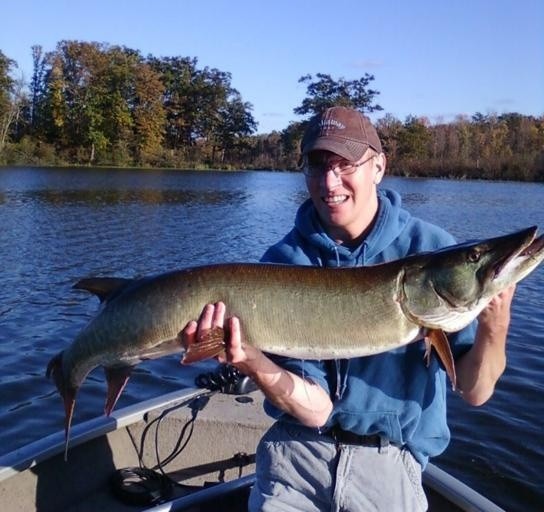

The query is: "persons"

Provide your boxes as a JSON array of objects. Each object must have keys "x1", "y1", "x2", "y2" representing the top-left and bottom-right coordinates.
[{"x1": 183, "y1": 107, "x2": 517, "y2": 512}]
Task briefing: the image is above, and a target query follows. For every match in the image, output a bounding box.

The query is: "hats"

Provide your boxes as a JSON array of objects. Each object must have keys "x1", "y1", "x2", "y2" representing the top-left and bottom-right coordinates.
[{"x1": 297, "y1": 105, "x2": 382, "y2": 168}]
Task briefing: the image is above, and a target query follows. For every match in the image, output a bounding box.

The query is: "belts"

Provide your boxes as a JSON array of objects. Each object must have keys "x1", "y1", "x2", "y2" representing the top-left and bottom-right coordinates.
[{"x1": 325, "y1": 428, "x2": 379, "y2": 448}]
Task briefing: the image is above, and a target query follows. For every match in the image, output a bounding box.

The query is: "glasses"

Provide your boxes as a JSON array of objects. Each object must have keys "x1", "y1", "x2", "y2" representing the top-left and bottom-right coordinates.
[{"x1": 301, "y1": 156, "x2": 376, "y2": 176}]
